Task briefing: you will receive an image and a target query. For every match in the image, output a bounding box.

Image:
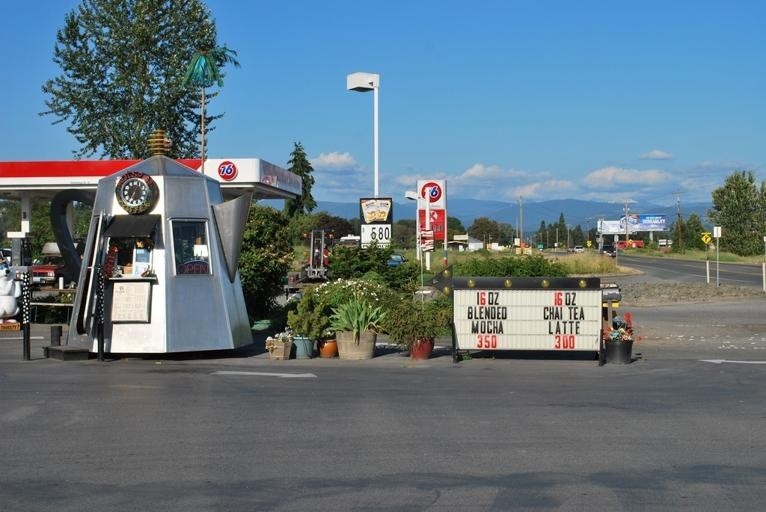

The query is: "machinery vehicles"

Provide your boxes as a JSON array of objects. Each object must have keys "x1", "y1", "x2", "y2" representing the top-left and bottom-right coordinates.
[{"x1": 299, "y1": 227, "x2": 336, "y2": 284}]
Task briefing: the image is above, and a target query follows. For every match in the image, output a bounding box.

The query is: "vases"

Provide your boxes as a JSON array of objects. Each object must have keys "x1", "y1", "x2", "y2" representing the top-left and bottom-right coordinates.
[
  {"x1": 266, "y1": 339, "x2": 292, "y2": 360},
  {"x1": 316, "y1": 339, "x2": 336, "y2": 358}
]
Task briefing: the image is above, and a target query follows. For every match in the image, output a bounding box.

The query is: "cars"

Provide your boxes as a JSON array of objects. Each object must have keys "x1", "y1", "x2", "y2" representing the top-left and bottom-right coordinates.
[
  {"x1": 387, "y1": 253, "x2": 408, "y2": 266},
  {"x1": 0, "y1": 235, "x2": 88, "y2": 287}
]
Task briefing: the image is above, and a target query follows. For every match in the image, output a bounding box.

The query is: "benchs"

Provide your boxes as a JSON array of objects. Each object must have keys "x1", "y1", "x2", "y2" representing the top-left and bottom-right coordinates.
[{"x1": 25, "y1": 302, "x2": 73, "y2": 325}]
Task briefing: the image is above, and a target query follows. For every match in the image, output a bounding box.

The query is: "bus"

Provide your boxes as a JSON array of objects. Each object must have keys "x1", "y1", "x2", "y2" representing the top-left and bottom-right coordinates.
[{"x1": 612, "y1": 240, "x2": 645, "y2": 250}]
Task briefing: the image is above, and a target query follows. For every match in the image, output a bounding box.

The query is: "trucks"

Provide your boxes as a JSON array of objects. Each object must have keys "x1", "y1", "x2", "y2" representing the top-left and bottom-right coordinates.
[
  {"x1": 340, "y1": 234, "x2": 360, "y2": 248},
  {"x1": 658, "y1": 239, "x2": 672, "y2": 248}
]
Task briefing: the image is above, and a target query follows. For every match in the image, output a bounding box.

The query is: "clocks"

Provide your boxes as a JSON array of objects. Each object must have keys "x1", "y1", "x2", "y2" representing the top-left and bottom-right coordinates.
[{"x1": 115, "y1": 170, "x2": 156, "y2": 214}]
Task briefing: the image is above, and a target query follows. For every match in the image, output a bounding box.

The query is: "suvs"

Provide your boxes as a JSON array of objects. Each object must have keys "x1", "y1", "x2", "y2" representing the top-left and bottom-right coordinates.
[{"x1": 600, "y1": 245, "x2": 617, "y2": 257}]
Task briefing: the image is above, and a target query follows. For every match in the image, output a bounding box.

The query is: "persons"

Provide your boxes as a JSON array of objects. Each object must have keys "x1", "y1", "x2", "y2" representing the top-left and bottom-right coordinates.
[{"x1": 126, "y1": 238, "x2": 152, "y2": 267}]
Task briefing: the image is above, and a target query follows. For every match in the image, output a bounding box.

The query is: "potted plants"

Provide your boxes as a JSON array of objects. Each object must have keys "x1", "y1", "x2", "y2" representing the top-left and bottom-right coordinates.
[
  {"x1": 330, "y1": 299, "x2": 388, "y2": 360},
  {"x1": 382, "y1": 299, "x2": 452, "y2": 362},
  {"x1": 285, "y1": 282, "x2": 329, "y2": 360}
]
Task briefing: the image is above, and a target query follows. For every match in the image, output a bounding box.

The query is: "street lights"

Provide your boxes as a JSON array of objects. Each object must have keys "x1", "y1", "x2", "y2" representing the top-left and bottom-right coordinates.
[
  {"x1": 671, "y1": 190, "x2": 683, "y2": 254},
  {"x1": 526, "y1": 213, "x2": 604, "y2": 252},
  {"x1": 516, "y1": 194, "x2": 524, "y2": 243},
  {"x1": 344, "y1": 73, "x2": 382, "y2": 200},
  {"x1": 405, "y1": 189, "x2": 431, "y2": 270},
  {"x1": 620, "y1": 198, "x2": 632, "y2": 241}
]
